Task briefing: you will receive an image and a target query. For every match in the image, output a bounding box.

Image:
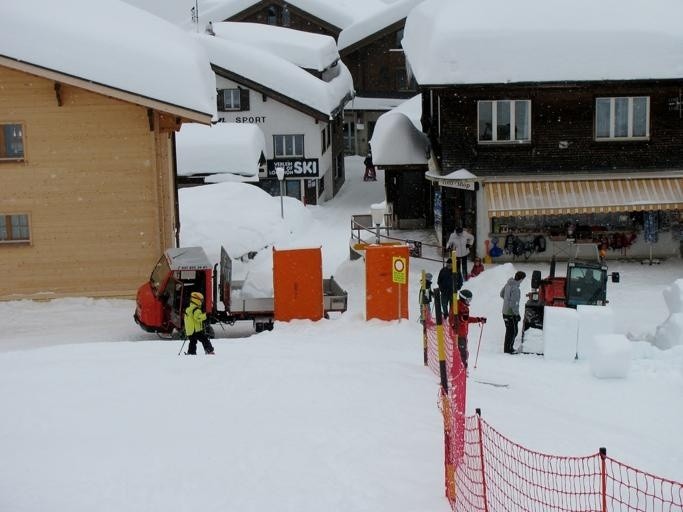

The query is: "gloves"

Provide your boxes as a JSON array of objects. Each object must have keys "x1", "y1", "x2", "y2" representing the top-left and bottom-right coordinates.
[
  {"x1": 463, "y1": 315, "x2": 468, "y2": 320},
  {"x1": 478, "y1": 317, "x2": 486, "y2": 323}
]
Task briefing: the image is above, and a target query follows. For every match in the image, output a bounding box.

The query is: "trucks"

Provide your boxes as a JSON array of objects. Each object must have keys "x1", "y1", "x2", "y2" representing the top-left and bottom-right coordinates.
[{"x1": 130, "y1": 245, "x2": 348, "y2": 341}]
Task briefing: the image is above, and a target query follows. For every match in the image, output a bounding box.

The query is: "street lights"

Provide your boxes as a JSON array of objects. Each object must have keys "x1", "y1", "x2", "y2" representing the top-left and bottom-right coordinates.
[
  {"x1": 371, "y1": 203, "x2": 387, "y2": 244},
  {"x1": 275, "y1": 167, "x2": 287, "y2": 221}
]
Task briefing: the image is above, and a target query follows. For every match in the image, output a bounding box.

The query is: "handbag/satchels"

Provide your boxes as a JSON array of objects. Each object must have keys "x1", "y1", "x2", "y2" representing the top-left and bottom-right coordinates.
[{"x1": 505, "y1": 234, "x2": 546, "y2": 255}]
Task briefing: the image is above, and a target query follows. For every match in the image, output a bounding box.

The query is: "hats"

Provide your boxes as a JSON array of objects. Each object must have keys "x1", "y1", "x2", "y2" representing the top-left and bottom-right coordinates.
[
  {"x1": 446, "y1": 258, "x2": 452, "y2": 264},
  {"x1": 455, "y1": 223, "x2": 463, "y2": 233}
]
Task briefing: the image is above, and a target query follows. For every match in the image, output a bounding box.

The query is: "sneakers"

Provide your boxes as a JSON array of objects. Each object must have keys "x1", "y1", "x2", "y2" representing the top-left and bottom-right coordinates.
[{"x1": 511, "y1": 350, "x2": 519, "y2": 354}]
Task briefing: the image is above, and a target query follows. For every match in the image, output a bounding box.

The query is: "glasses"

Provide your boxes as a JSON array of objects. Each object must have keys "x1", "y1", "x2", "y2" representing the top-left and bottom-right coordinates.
[{"x1": 459, "y1": 293, "x2": 471, "y2": 303}]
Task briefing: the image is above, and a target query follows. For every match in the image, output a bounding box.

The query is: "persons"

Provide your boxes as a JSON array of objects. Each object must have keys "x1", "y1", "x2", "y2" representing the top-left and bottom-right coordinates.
[
  {"x1": 418, "y1": 272, "x2": 434, "y2": 323},
  {"x1": 436, "y1": 257, "x2": 463, "y2": 318},
  {"x1": 499, "y1": 270, "x2": 525, "y2": 353},
  {"x1": 183, "y1": 291, "x2": 215, "y2": 355},
  {"x1": 465, "y1": 256, "x2": 484, "y2": 280},
  {"x1": 445, "y1": 223, "x2": 473, "y2": 277},
  {"x1": 363, "y1": 150, "x2": 376, "y2": 181},
  {"x1": 447, "y1": 289, "x2": 487, "y2": 377}
]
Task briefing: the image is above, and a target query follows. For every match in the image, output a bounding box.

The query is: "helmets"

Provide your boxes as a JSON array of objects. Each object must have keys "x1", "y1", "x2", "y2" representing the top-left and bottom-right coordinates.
[
  {"x1": 425, "y1": 274, "x2": 433, "y2": 282},
  {"x1": 190, "y1": 292, "x2": 204, "y2": 306},
  {"x1": 459, "y1": 290, "x2": 472, "y2": 303},
  {"x1": 474, "y1": 257, "x2": 481, "y2": 267}
]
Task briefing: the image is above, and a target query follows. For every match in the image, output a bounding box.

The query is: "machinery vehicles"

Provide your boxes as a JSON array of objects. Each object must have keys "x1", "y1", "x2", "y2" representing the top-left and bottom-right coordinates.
[{"x1": 518, "y1": 242, "x2": 621, "y2": 358}]
